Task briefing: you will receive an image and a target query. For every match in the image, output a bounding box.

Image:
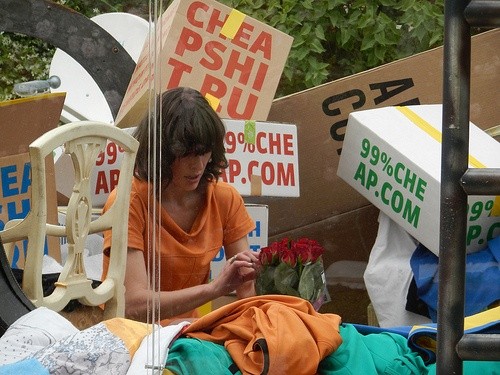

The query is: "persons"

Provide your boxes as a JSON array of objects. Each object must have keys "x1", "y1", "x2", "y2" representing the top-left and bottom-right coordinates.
[{"x1": 99, "y1": 86, "x2": 260, "y2": 326}]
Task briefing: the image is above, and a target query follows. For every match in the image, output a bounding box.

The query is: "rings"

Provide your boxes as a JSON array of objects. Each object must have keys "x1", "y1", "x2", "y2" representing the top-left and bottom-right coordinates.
[{"x1": 229, "y1": 255, "x2": 239, "y2": 264}]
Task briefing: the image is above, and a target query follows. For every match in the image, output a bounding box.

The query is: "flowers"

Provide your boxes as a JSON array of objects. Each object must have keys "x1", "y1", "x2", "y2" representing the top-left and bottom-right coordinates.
[{"x1": 254, "y1": 238, "x2": 326, "y2": 312}]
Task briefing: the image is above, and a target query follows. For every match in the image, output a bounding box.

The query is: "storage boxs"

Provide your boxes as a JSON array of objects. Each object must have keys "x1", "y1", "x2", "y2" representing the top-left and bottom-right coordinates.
[
  {"x1": 337, "y1": 104, "x2": 500, "y2": 258},
  {"x1": 0, "y1": 92, "x2": 67, "y2": 270},
  {"x1": 115, "y1": 0, "x2": 294, "y2": 129},
  {"x1": 90, "y1": 118, "x2": 300, "y2": 207},
  {"x1": 210, "y1": 204, "x2": 268, "y2": 295}
]
